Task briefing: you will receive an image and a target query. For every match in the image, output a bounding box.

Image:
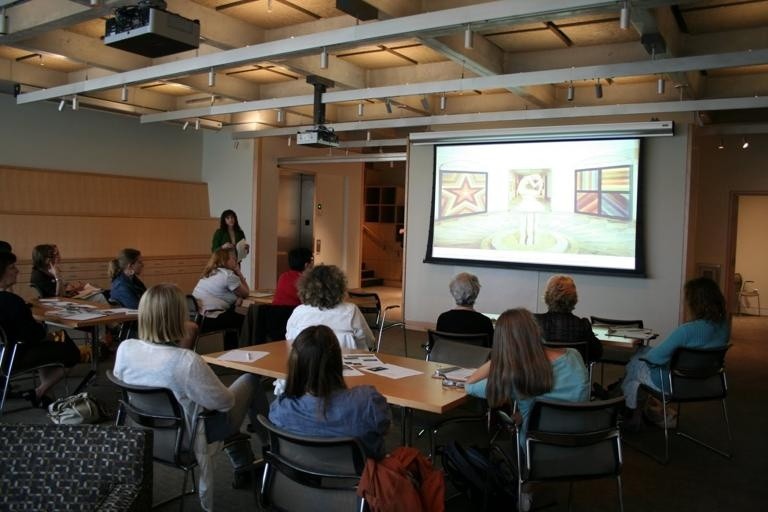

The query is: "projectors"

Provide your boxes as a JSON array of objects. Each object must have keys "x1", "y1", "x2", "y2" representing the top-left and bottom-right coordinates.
[
  {"x1": 296, "y1": 132, "x2": 339, "y2": 148},
  {"x1": 103, "y1": 7, "x2": 200, "y2": 59}
]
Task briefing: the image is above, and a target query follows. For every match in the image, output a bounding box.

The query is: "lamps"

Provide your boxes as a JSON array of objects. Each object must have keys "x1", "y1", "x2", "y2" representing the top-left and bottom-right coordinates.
[
  {"x1": 621, "y1": 3, "x2": 628, "y2": 31},
  {"x1": 321, "y1": 47, "x2": 327, "y2": 69},
  {"x1": 358, "y1": 100, "x2": 363, "y2": 116},
  {"x1": 595, "y1": 78, "x2": 603, "y2": 100},
  {"x1": 658, "y1": 75, "x2": 664, "y2": 94},
  {"x1": 379, "y1": 146, "x2": 392, "y2": 167},
  {"x1": 58, "y1": 100, "x2": 65, "y2": 113},
  {"x1": 181, "y1": 120, "x2": 189, "y2": 132},
  {"x1": 208, "y1": 66, "x2": 214, "y2": 87},
  {"x1": 717, "y1": 134, "x2": 724, "y2": 150},
  {"x1": 287, "y1": 135, "x2": 294, "y2": 146},
  {"x1": 465, "y1": 22, "x2": 473, "y2": 49},
  {"x1": 385, "y1": 97, "x2": 391, "y2": 112},
  {"x1": 440, "y1": 92, "x2": 445, "y2": 110},
  {"x1": 421, "y1": 94, "x2": 428, "y2": 111},
  {"x1": 277, "y1": 107, "x2": 283, "y2": 122},
  {"x1": 366, "y1": 129, "x2": 372, "y2": 141},
  {"x1": 234, "y1": 141, "x2": 239, "y2": 148},
  {"x1": 329, "y1": 148, "x2": 351, "y2": 155},
  {"x1": 72, "y1": 93, "x2": 78, "y2": 111},
  {"x1": 122, "y1": 84, "x2": 128, "y2": 101},
  {"x1": 740, "y1": 134, "x2": 751, "y2": 151},
  {"x1": 568, "y1": 81, "x2": 575, "y2": 102},
  {"x1": 196, "y1": 116, "x2": 201, "y2": 132}
]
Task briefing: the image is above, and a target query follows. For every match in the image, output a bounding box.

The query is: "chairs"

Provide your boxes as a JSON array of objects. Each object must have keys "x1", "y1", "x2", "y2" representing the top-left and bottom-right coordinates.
[
  {"x1": 0, "y1": 424, "x2": 154, "y2": 512},
  {"x1": 106, "y1": 368, "x2": 264, "y2": 510},
  {"x1": 488, "y1": 397, "x2": 624, "y2": 510},
  {"x1": 346, "y1": 291, "x2": 408, "y2": 357},
  {"x1": 98, "y1": 289, "x2": 296, "y2": 345},
  {"x1": 735, "y1": 273, "x2": 760, "y2": 315},
  {"x1": 0, "y1": 325, "x2": 69, "y2": 414},
  {"x1": 617, "y1": 347, "x2": 734, "y2": 465},
  {"x1": 420, "y1": 316, "x2": 660, "y2": 397},
  {"x1": 256, "y1": 413, "x2": 423, "y2": 510}
]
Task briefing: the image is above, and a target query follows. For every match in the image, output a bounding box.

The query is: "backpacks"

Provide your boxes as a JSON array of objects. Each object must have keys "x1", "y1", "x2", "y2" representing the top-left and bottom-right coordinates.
[{"x1": 433, "y1": 437, "x2": 519, "y2": 511}]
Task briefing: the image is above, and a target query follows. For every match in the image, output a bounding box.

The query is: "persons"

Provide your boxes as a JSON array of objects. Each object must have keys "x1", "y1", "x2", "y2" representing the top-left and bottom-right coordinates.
[
  {"x1": 284, "y1": 264, "x2": 377, "y2": 354},
  {"x1": 0, "y1": 253, "x2": 81, "y2": 409},
  {"x1": 271, "y1": 249, "x2": 314, "y2": 306},
  {"x1": 268, "y1": 325, "x2": 389, "y2": 465},
  {"x1": 113, "y1": 285, "x2": 267, "y2": 490},
  {"x1": 437, "y1": 271, "x2": 494, "y2": 346},
  {"x1": 107, "y1": 248, "x2": 197, "y2": 350},
  {"x1": 31, "y1": 245, "x2": 122, "y2": 352},
  {"x1": 618, "y1": 276, "x2": 729, "y2": 435},
  {"x1": 212, "y1": 210, "x2": 249, "y2": 275},
  {"x1": 441, "y1": 310, "x2": 590, "y2": 511},
  {"x1": 532, "y1": 274, "x2": 603, "y2": 366},
  {"x1": 191, "y1": 243, "x2": 249, "y2": 351}
]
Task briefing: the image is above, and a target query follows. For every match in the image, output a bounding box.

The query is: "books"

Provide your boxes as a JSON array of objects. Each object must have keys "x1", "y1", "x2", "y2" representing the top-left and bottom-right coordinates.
[
  {"x1": 434, "y1": 366, "x2": 476, "y2": 382},
  {"x1": 37, "y1": 284, "x2": 139, "y2": 320},
  {"x1": 248, "y1": 292, "x2": 272, "y2": 298},
  {"x1": 236, "y1": 238, "x2": 247, "y2": 263},
  {"x1": 593, "y1": 323, "x2": 657, "y2": 342}
]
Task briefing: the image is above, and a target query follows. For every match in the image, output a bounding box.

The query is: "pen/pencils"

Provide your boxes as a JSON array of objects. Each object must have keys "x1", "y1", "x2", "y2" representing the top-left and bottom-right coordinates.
[{"x1": 248, "y1": 353, "x2": 253, "y2": 361}]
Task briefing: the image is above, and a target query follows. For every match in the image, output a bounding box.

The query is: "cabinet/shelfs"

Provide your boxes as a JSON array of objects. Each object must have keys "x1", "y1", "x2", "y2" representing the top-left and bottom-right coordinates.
[{"x1": 364, "y1": 185, "x2": 403, "y2": 286}]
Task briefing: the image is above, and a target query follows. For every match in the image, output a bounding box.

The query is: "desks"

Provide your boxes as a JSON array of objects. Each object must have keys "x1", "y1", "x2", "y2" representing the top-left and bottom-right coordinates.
[
  {"x1": 25, "y1": 297, "x2": 139, "y2": 371},
  {"x1": 199, "y1": 341, "x2": 480, "y2": 472}
]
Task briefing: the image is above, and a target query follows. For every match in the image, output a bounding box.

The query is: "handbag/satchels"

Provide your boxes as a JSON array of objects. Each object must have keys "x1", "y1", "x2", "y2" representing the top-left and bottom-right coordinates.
[
  {"x1": 48, "y1": 392, "x2": 105, "y2": 425},
  {"x1": 642, "y1": 393, "x2": 679, "y2": 432}
]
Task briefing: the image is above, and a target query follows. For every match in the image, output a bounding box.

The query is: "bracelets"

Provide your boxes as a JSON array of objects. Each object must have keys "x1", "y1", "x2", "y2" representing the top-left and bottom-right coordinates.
[
  {"x1": 55, "y1": 277, "x2": 62, "y2": 281},
  {"x1": 242, "y1": 277, "x2": 246, "y2": 282}
]
